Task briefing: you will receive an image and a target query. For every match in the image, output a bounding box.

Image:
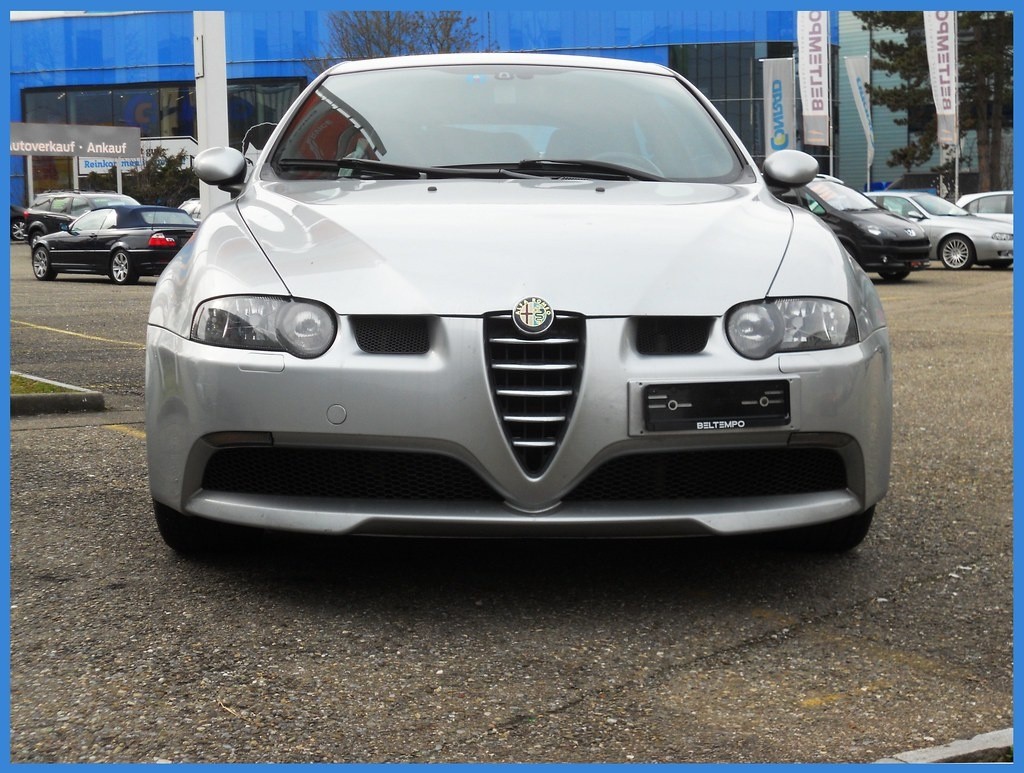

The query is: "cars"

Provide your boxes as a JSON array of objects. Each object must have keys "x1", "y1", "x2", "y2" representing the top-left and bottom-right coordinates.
[
  {"x1": 10, "y1": 205, "x2": 28, "y2": 241},
  {"x1": 950, "y1": 193, "x2": 1014, "y2": 227},
  {"x1": 30, "y1": 204, "x2": 202, "y2": 285},
  {"x1": 178, "y1": 198, "x2": 202, "y2": 222},
  {"x1": 863, "y1": 191, "x2": 1014, "y2": 270},
  {"x1": 761, "y1": 173, "x2": 932, "y2": 282},
  {"x1": 146, "y1": 48, "x2": 893, "y2": 569}
]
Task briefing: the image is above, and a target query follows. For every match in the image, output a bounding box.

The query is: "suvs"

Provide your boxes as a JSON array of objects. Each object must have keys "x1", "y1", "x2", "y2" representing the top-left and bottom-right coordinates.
[{"x1": 24, "y1": 189, "x2": 141, "y2": 250}]
